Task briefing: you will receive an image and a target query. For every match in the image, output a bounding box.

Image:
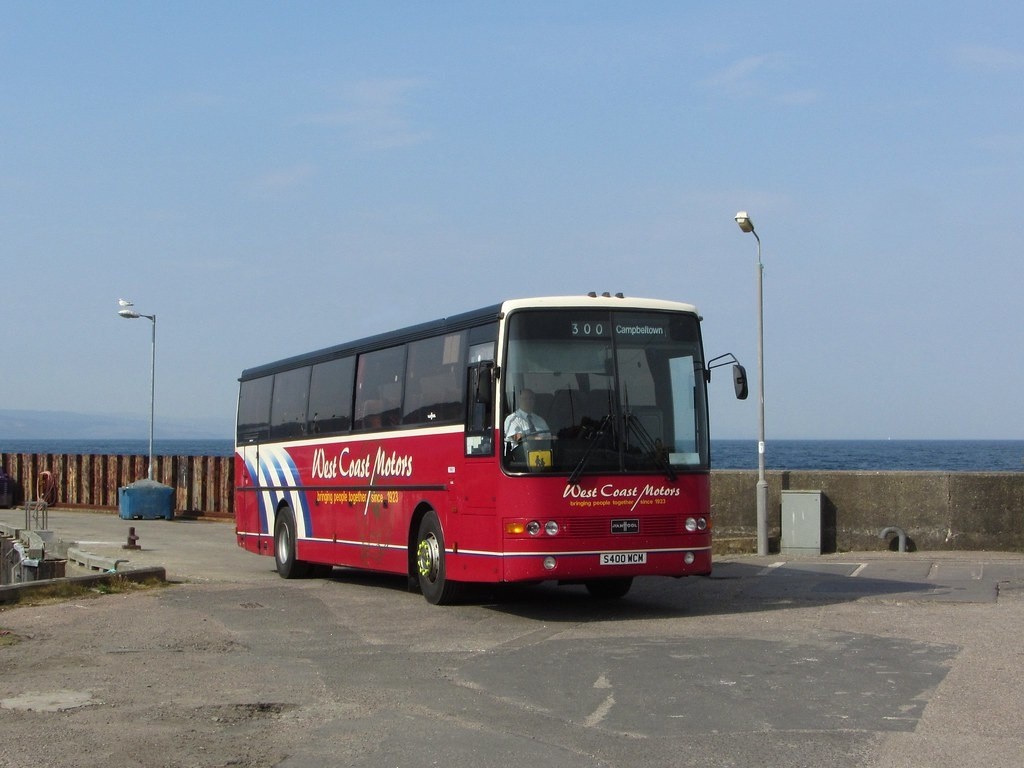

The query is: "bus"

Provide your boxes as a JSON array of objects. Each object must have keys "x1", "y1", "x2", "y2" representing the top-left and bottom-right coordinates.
[{"x1": 232, "y1": 291, "x2": 750, "y2": 608}]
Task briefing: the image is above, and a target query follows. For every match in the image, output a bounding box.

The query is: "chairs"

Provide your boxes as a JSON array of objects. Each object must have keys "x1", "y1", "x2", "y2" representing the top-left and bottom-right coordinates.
[{"x1": 361, "y1": 399, "x2": 383, "y2": 430}]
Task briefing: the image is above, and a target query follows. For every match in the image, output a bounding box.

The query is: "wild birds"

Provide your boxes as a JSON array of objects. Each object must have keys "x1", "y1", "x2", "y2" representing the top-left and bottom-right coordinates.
[{"x1": 118, "y1": 298, "x2": 134, "y2": 311}]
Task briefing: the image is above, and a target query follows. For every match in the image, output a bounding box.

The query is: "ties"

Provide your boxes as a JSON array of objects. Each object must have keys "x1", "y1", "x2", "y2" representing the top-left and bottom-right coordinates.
[{"x1": 526, "y1": 415, "x2": 538, "y2": 437}]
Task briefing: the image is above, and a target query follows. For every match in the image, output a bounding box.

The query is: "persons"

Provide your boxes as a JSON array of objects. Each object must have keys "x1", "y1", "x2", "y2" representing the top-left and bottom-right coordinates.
[{"x1": 504, "y1": 389, "x2": 551, "y2": 456}]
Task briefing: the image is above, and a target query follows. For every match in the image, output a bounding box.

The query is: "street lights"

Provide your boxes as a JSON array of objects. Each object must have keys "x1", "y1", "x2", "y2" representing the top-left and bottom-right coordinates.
[
  {"x1": 733, "y1": 210, "x2": 770, "y2": 554},
  {"x1": 119, "y1": 309, "x2": 158, "y2": 477}
]
